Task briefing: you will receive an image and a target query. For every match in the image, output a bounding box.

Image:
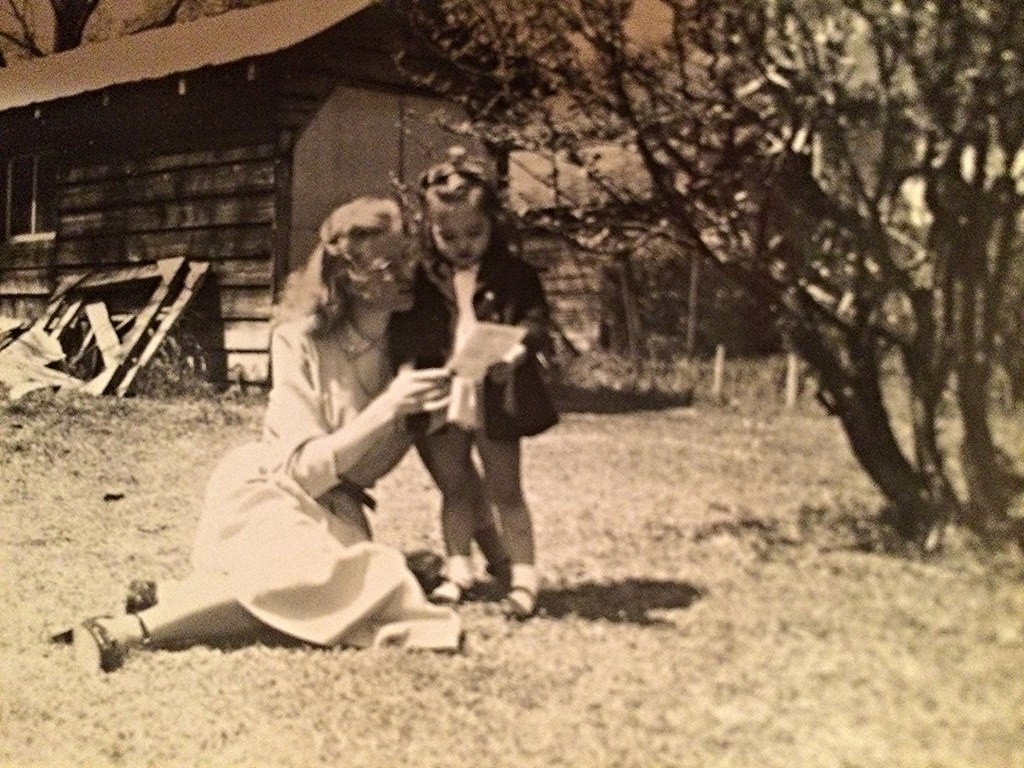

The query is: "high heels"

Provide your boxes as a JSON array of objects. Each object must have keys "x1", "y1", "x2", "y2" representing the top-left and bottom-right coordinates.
[
  {"x1": 51, "y1": 610, "x2": 153, "y2": 672},
  {"x1": 124, "y1": 577, "x2": 158, "y2": 614}
]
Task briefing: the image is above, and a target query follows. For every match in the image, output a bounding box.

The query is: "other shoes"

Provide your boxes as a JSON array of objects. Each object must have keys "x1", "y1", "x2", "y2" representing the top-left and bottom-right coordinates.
[
  {"x1": 429, "y1": 575, "x2": 479, "y2": 606},
  {"x1": 496, "y1": 585, "x2": 538, "y2": 619}
]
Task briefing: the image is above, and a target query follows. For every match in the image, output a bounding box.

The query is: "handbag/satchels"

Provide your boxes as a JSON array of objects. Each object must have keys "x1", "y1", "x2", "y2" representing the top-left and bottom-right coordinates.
[{"x1": 526, "y1": 320, "x2": 579, "y2": 383}]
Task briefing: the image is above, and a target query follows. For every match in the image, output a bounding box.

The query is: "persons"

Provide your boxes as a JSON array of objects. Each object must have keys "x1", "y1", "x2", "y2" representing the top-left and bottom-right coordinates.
[
  {"x1": 385, "y1": 154, "x2": 552, "y2": 619},
  {"x1": 48, "y1": 197, "x2": 455, "y2": 680}
]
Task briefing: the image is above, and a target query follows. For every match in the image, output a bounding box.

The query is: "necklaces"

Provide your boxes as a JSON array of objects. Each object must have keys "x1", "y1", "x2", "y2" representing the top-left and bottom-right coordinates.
[{"x1": 339, "y1": 346, "x2": 376, "y2": 400}]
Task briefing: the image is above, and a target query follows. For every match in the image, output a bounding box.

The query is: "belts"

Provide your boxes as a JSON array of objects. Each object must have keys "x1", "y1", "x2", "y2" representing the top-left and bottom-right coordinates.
[{"x1": 335, "y1": 475, "x2": 375, "y2": 509}]
